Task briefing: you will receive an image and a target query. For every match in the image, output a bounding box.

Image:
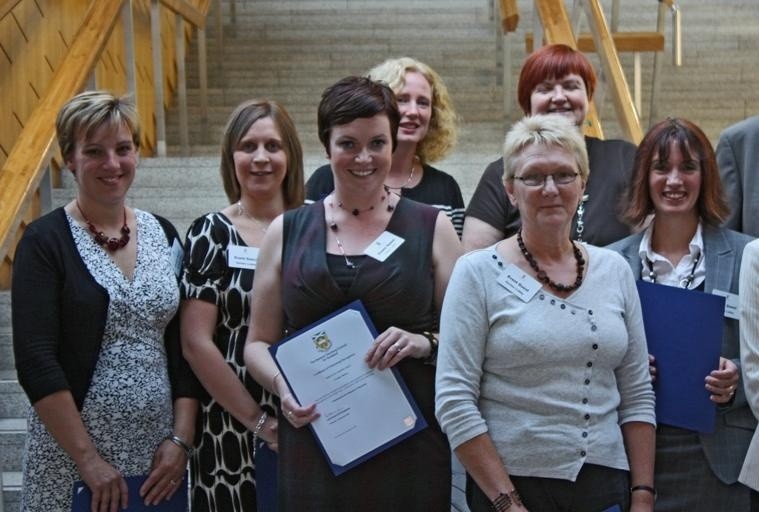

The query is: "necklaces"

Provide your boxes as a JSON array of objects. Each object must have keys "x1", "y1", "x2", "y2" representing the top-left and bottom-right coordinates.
[
  {"x1": 387, "y1": 157, "x2": 416, "y2": 189},
  {"x1": 517, "y1": 226, "x2": 585, "y2": 292},
  {"x1": 646, "y1": 251, "x2": 701, "y2": 289},
  {"x1": 237, "y1": 200, "x2": 267, "y2": 234},
  {"x1": 329, "y1": 185, "x2": 394, "y2": 271},
  {"x1": 75, "y1": 198, "x2": 130, "y2": 251}
]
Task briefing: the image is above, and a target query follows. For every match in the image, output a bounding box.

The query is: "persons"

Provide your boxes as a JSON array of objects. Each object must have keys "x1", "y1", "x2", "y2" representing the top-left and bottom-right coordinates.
[
  {"x1": 461, "y1": 41, "x2": 654, "y2": 257},
  {"x1": 179, "y1": 96, "x2": 318, "y2": 512},
  {"x1": 715, "y1": 115, "x2": 759, "y2": 239},
  {"x1": 430, "y1": 112, "x2": 660, "y2": 512},
  {"x1": 241, "y1": 73, "x2": 463, "y2": 511},
  {"x1": 600, "y1": 115, "x2": 758, "y2": 512},
  {"x1": 732, "y1": 237, "x2": 759, "y2": 512},
  {"x1": 9, "y1": 89, "x2": 198, "y2": 511},
  {"x1": 304, "y1": 57, "x2": 466, "y2": 333}
]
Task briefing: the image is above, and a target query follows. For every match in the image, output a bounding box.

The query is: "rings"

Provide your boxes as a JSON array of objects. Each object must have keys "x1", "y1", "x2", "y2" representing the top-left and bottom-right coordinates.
[
  {"x1": 730, "y1": 385, "x2": 735, "y2": 397},
  {"x1": 169, "y1": 479, "x2": 177, "y2": 485},
  {"x1": 394, "y1": 342, "x2": 402, "y2": 352},
  {"x1": 287, "y1": 411, "x2": 293, "y2": 418}
]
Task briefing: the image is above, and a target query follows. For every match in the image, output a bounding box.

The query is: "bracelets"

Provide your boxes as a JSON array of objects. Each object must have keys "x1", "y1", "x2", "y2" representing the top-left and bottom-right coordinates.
[
  {"x1": 490, "y1": 489, "x2": 523, "y2": 512},
  {"x1": 169, "y1": 433, "x2": 190, "y2": 454},
  {"x1": 422, "y1": 331, "x2": 439, "y2": 363},
  {"x1": 255, "y1": 412, "x2": 267, "y2": 433},
  {"x1": 630, "y1": 485, "x2": 657, "y2": 495},
  {"x1": 272, "y1": 372, "x2": 280, "y2": 394}
]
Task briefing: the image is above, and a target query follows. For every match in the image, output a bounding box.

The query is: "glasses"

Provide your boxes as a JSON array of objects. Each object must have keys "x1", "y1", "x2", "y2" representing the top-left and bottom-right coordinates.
[{"x1": 510, "y1": 170, "x2": 583, "y2": 188}]
